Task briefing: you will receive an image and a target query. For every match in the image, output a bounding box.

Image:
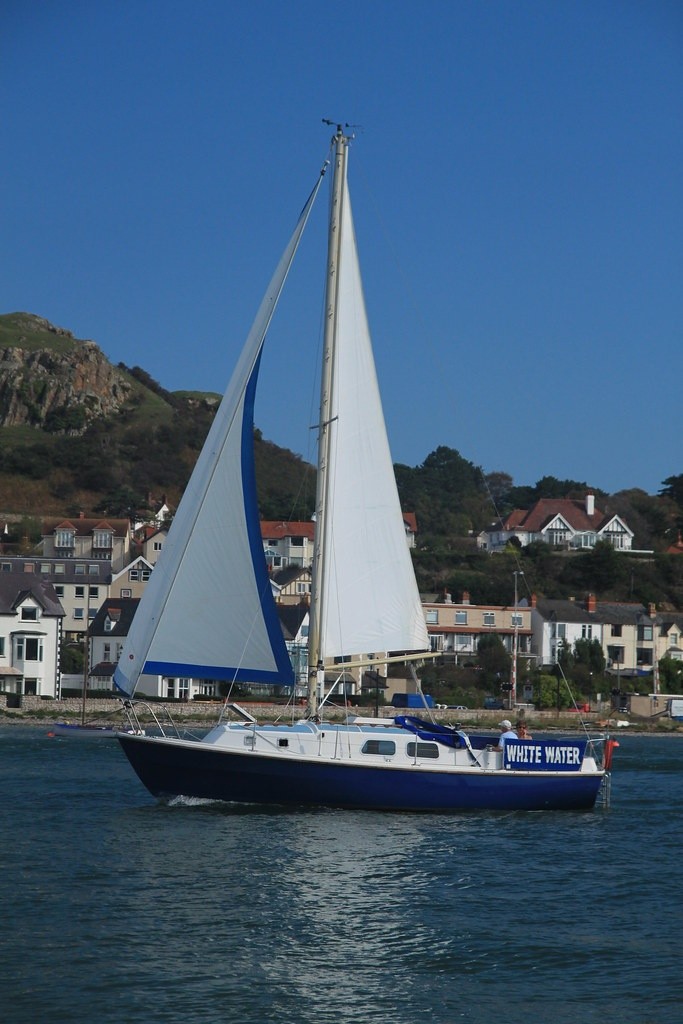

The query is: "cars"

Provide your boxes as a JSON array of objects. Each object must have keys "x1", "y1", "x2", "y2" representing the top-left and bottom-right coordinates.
[{"x1": 445, "y1": 706, "x2": 468, "y2": 709}]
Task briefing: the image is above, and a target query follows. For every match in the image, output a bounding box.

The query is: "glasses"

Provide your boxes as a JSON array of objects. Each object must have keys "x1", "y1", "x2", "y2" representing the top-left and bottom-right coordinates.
[{"x1": 518, "y1": 725, "x2": 526, "y2": 730}]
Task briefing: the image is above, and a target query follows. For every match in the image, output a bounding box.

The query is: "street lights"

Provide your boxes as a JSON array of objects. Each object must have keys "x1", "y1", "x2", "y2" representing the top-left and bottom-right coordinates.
[{"x1": 513, "y1": 570, "x2": 523, "y2": 707}]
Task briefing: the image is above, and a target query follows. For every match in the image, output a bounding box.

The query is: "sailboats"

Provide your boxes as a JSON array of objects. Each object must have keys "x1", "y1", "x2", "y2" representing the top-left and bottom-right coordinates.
[{"x1": 123, "y1": 118, "x2": 620, "y2": 817}]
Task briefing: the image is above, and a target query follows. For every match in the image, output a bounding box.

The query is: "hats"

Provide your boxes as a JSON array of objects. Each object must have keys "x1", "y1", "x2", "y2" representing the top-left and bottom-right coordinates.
[{"x1": 498, "y1": 719, "x2": 511, "y2": 729}]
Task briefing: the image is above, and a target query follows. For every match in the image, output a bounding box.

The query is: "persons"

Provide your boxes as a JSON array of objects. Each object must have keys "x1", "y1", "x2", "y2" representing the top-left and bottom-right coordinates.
[
  {"x1": 484, "y1": 720, "x2": 521, "y2": 755},
  {"x1": 513, "y1": 719, "x2": 533, "y2": 742}
]
